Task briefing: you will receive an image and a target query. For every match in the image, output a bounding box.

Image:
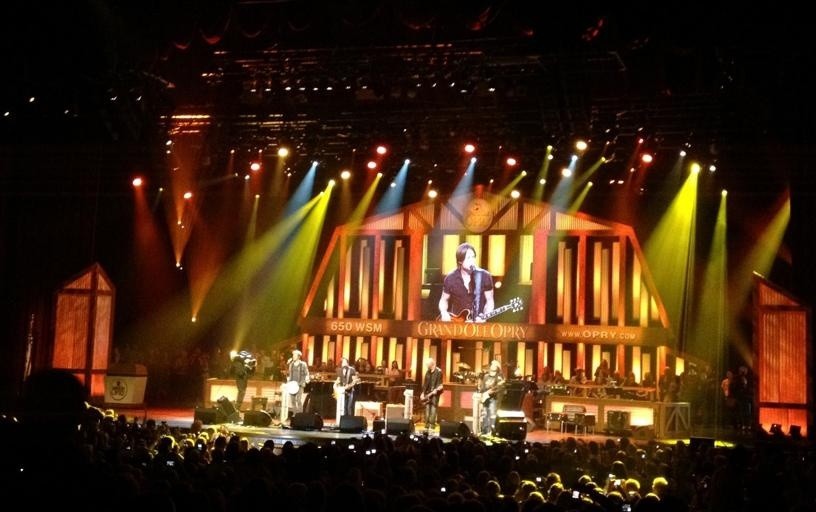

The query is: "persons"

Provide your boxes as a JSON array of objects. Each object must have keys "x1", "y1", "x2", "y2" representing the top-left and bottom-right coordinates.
[
  {"x1": 257, "y1": 346, "x2": 404, "y2": 386},
  {"x1": 283, "y1": 350, "x2": 310, "y2": 417},
  {"x1": 473, "y1": 360, "x2": 505, "y2": 434},
  {"x1": 90, "y1": 404, "x2": 815, "y2": 512},
  {"x1": 420, "y1": 357, "x2": 444, "y2": 434},
  {"x1": 233, "y1": 350, "x2": 257, "y2": 410},
  {"x1": 112, "y1": 339, "x2": 233, "y2": 404},
  {"x1": 438, "y1": 242, "x2": 495, "y2": 324},
  {"x1": 332, "y1": 357, "x2": 360, "y2": 416},
  {"x1": 539, "y1": 358, "x2": 754, "y2": 433}
]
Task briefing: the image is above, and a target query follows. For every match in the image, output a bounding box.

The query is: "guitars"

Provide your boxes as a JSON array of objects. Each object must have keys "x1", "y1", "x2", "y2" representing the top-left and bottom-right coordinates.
[
  {"x1": 479, "y1": 378, "x2": 507, "y2": 403},
  {"x1": 435, "y1": 297, "x2": 523, "y2": 323},
  {"x1": 418, "y1": 384, "x2": 443, "y2": 407},
  {"x1": 332, "y1": 378, "x2": 361, "y2": 399}
]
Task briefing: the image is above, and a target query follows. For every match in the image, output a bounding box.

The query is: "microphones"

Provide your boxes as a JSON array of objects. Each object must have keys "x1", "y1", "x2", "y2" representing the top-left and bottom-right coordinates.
[
  {"x1": 287, "y1": 358, "x2": 293, "y2": 364},
  {"x1": 469, "y1": 266, "x2": 477, "y2": 273}
]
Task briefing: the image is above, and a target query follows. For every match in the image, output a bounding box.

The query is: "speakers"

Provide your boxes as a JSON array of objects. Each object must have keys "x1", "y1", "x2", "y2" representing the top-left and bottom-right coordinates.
[
  {"x1": 194, "y1": 407, "x2": 217, "y2": 424},
  {"x1": 340, "y1": 416, "x2": 367, "y2": 433},
  {"x1": 388, "y1": 418, "x2": 415, "y2": 434},
  {"x1": 440, "y1": 421, "x2": 470, "y2": 438},
  {"x1": 294, "y1": 413, "x2": 323, "y2": 430},
  {"x1": 243, "y1": 409, "x2": 272, "y2": 426},
  {"x1": 689, "y1": 437, "x2": 716, "y2": 447}
]
